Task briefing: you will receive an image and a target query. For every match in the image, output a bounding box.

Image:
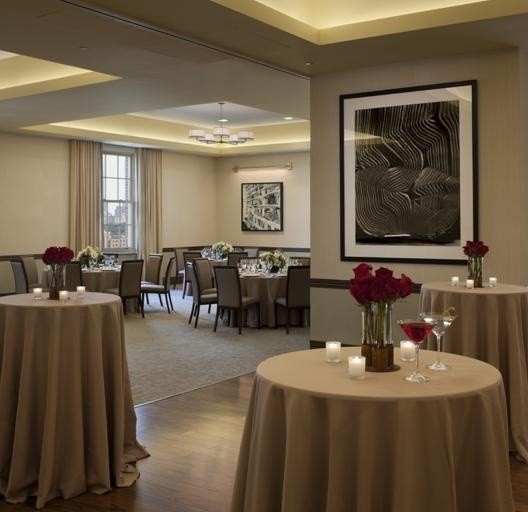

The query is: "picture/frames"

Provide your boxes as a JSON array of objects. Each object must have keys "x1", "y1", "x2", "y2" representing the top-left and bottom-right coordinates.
[
  {"x1": 240, "y1": 182, "x2": 285, "y2": 232},
  {"x1": 339, "y1": 81, "x2": 481, "y2": 265}
]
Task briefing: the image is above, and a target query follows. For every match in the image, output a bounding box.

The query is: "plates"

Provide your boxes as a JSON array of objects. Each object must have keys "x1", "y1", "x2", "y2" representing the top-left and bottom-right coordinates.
[
  {"x1": 244, "y1": 273, "x2": 260, "y2": 277},
  {"x1": 277, "y1": 274, "x2": 287, "y2": 277},
  {"x1": 100, "y1": 268, "x2": 117, "y2": 271}
]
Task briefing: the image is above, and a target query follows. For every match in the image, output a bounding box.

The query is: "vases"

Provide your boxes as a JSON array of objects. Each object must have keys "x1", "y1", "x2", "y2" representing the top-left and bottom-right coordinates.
[
  {"x1": 467, "y1": 256, "x2": 483, "y2": 284},
  {"x1": 357, "y1": 310, "x2": 396, "y2": 369}
]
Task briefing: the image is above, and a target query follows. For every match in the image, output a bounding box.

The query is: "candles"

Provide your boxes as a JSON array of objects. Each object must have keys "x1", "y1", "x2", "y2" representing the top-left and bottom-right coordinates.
[
  {"x1": 326, "y1": 339, "x2": 340, "y2": 361},
  {"x1": 466, "y1": 280, "x2": 474, "y2": 287},
  {"x1": 490, "y1": 277, "x2": 497, "y2": 285},
  {"x1": 451, "y1": 276, "x2": 459, "y2": 284},
  {"x1": 400, "y1": 340, "x2": 414, "y2": 361}
]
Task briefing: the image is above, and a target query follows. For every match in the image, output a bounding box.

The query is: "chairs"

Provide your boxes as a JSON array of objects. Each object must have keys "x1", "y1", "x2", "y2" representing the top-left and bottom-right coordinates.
[
  {"x1": 141, "y1": 256, "x2": 176, "y2": 314},
  {"x1": 182, "y1": 251, "x2": 202, "y2": 299},
  {"x1": 274, "y1": 265, "x2": 311, "y2": 334},
  {"x1": 289, "y1": 256, "x2": 311, "y2": 265},
  {"x1": 140, "y1": 254, "x2": 164, "y2": 307},
  {"x1": 189, "y1": 246, "x2": 212, "y2": 251},
  {"x1": 212, "y1": 265, "x2": 261, "y2": 336},
  {"x1": 9, "y1": 258, "x2": 30, "y2": 295},
  {"x1": 33, "y1": 256, "x2": 49, "y2": 285},
  {"x1": 66, "y1": 262, "x2": 82, "y2": 289},
  {"x1": 240, "y1": 257, "x2": 264, "y2": 264},
  {"x1": 191, "y1": 258, "x2": 217, "y2": 316},
  {"x1": 162, "y1": 248, "x2": 184, "y2": 289},
  {"x1": 184, "y1": 262, "x2": 217, "y2": 328},
  {"x1": 227, "y1": 251, "x2": 248, "y2": 267},
  {"x1": 102, "y1": 259, "x2": 145, "y2": 320}
]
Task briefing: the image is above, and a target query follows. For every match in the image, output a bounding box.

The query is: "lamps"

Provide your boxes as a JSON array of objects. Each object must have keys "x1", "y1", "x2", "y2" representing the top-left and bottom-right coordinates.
[{"x1": 190, "y1": 102, "x2": 252, "y2": 146}]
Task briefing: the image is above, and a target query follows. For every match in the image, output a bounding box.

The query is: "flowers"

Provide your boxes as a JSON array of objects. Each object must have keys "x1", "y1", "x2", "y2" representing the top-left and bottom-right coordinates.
[
  {"x1": 347, "y1": 263, "x2": 417, "y2": 348},
  {"x1": 462, "y1": 240, "x2": 489, "y2": 274}
]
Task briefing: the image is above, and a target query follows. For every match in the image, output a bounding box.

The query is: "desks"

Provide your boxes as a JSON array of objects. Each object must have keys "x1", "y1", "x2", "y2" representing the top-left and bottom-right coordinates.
[
  {"x1": 1, "y1": 292, "x2": 150, "y2": 506},
  {"x1": 231, "y1": 344, "x2": 514, "y2": 510},
  {"x1": 424, "y1": 281, "x2": 528, "y2": 460}
]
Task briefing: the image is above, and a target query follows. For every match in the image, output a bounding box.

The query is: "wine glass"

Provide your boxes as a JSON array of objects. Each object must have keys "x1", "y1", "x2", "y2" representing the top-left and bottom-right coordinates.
[
  {"x1": 87, "y1": 254, "x2": 115, "y2": 269},
  {"x1": 418, "y1": 311, "x2": 457, "y2": 371},
  {"x1": 239, "y1": 258, "x2": 299, "y2": 273},
  {"x1": 201, "y1": 247, "x2": 222, "y2": 260},
  {"x1": 394, "y1": 319, "x2": 434, "y2": 383}
]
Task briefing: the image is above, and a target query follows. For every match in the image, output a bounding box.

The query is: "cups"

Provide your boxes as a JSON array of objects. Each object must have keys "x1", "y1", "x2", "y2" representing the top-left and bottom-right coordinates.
[
  {"x1": 32, "y1": 287, "x2": 43, "y2": 300},
  {"x1": 326, "y1": 341, "x2": 341, "y2": 362},
  {"x1": 76, "y1": 285, "x2": 86, "y2": 298},
  {"x1": 451, "y1": 276, "x2": 460, "y2": 286},
  {"x1": 489, "y1": 276, "x2": 497, "y2": 288},
  {"x1": 400, "y1": 340, "x2": 416, "y2": 362},
  {"x1": 466, "y1": 279, "x2": 475, "y2": 289},
  {"x1": 59, "y1": 290, "x2": 68, "y2": 301}
]
